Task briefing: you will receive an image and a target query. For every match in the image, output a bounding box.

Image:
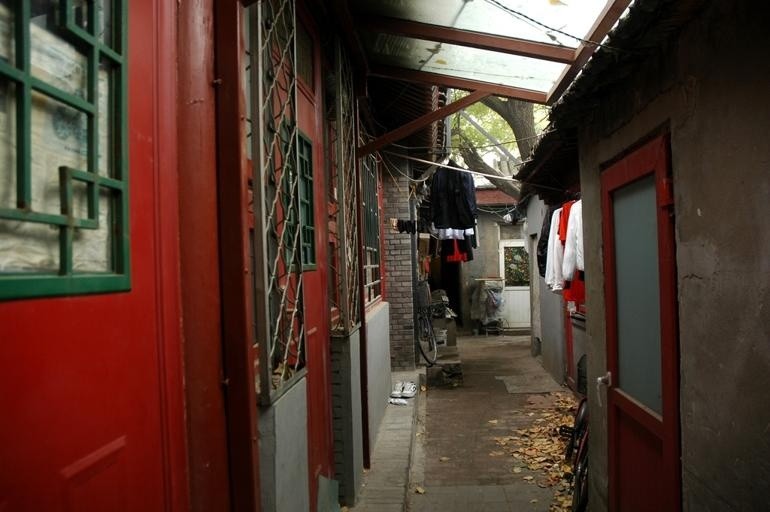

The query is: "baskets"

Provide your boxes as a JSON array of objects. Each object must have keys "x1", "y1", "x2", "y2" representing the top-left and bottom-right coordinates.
[
  {"x1": 577, "y1": 354, "x2": 587, "y2": 395},
  {"x1": 416, "y1": 285, "x2": 429, "y2": 307}
]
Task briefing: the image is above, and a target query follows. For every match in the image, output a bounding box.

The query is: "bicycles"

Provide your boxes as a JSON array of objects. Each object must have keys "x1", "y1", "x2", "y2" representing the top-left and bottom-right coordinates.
[
  {"x1": 559, "y1": 309, "x2": 588, "y2": 511},
  {"x1": 416, "y1": 279, "x2": 438, "y2": 365}
]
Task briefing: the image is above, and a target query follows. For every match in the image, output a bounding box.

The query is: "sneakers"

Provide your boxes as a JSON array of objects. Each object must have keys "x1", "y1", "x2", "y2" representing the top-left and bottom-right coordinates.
[
  {"x1": 390, "y1": 381, "x2": 403, "y2": 398},
  {"x1": 401, "y1": 380, "x2": 417, "y2": 397}
]
